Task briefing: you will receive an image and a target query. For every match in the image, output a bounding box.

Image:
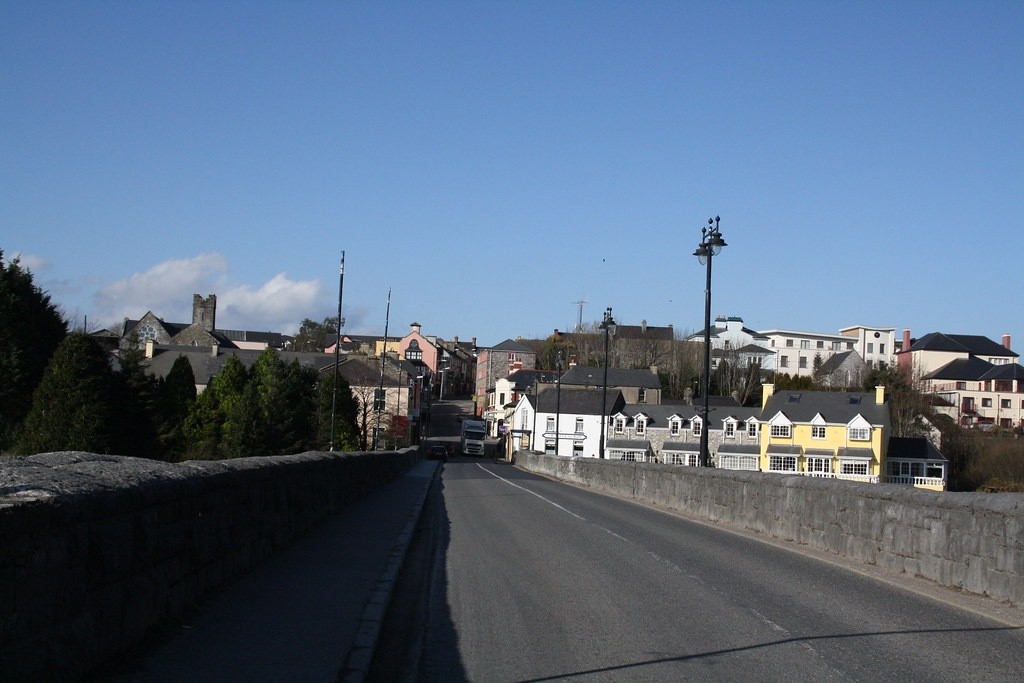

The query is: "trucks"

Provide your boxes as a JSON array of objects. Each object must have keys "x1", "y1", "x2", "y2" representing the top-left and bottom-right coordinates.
[{"x1": 461, "y1": 417, "x2": 487, "y2": 457}]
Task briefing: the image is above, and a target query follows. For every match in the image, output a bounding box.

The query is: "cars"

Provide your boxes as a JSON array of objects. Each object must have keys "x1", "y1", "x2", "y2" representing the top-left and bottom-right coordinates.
[{"x1": 430, "y1": 443, "x2": 455, "y2": 462}]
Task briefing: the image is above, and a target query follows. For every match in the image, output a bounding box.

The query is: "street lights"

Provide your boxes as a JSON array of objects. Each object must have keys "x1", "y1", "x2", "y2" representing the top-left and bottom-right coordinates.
[
  {"x1": 598, "y1": 307, "x2": 617, "y2": 458},
  {"x1": 691, "y1": 214, "x2": 728, "y2": 466},
  {"x1": 555, "y1": 348, "x2": 566, "y2": 456}
]
